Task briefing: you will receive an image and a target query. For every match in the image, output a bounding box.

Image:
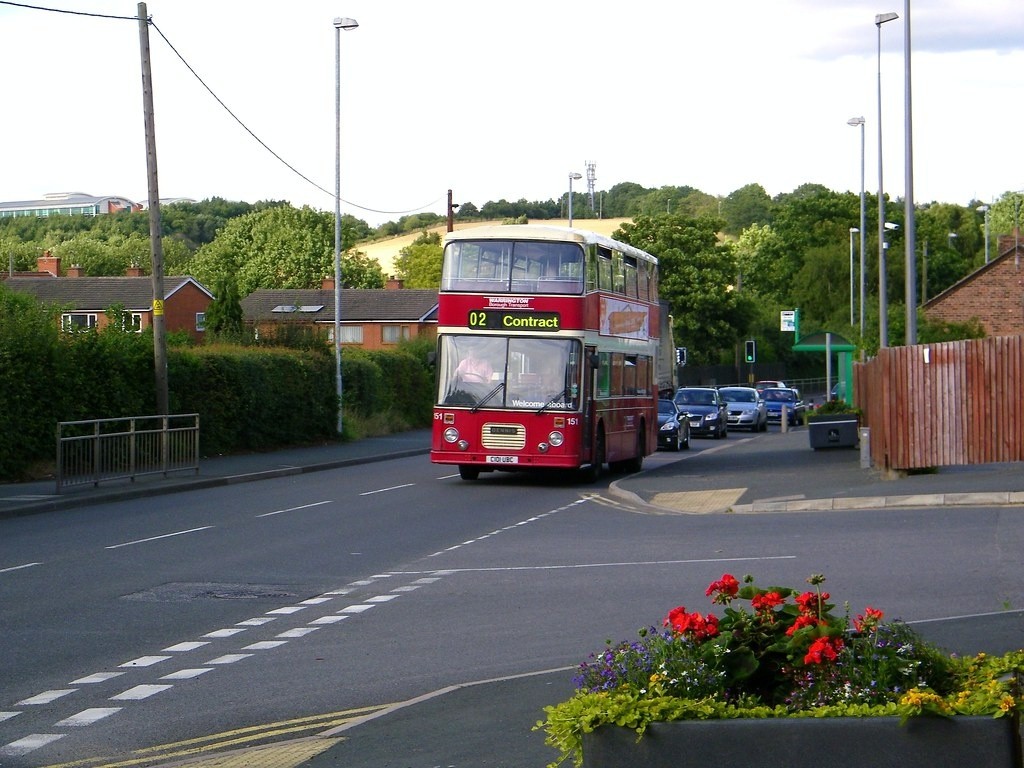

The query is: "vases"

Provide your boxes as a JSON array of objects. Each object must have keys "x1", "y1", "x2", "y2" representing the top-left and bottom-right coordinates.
[
  {"x1": 807, "y1": 414, "x2": 857, "y2": 423},
  {"x1": 583, "y1": 669, "x2": 1021, "y2": 767}
]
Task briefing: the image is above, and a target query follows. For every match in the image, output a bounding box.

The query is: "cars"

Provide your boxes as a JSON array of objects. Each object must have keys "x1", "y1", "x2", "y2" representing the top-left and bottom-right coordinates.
[
  {"x1": 827, "y1": 382, "x2": 847, "y2": 400},
  {"x1": 760, "y1": 388, "x2": 806, "y2": 427},
  {"x1": 656, "y1": 398, "x2": 691, "y2": 452},
  {"x1": 719, "y1": 387, "x2": 768, "y2": 434}
]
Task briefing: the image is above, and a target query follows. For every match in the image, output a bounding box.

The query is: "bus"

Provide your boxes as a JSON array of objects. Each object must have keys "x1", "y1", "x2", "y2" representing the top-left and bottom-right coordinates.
[{"x1": 431, "y1": 225, "x2": 661, "y2": 484}]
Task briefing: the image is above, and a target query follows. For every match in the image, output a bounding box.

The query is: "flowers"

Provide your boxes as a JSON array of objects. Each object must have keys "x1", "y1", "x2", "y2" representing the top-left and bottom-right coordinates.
[
  {"x1": 532, "y1": 575, "x2": 1024, "y2": 768},
  {"x1": 803, "y1": 400, "x2": 865, "y2": 432}
]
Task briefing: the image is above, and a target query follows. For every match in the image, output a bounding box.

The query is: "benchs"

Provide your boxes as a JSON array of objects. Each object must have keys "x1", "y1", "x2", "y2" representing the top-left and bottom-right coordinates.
[{"x1": 453, "y1": 277, "x2": 583, "y2": 294}]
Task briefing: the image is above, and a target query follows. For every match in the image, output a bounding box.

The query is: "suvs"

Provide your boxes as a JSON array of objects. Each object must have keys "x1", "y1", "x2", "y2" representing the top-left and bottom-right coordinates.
[
  {"x1": 673, "y1": 386, "x2": 728, "y2": 439},
  {"x1": 755, "y1": 381, "x2": 792, "y2": 400}
]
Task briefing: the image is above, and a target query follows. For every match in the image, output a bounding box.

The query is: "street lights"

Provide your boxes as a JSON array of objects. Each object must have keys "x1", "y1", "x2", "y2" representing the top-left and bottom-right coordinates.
[
  {"x1": 947, "y1": 232, "x2": 957, "y2": 261},
  {"x1": 850, "y1": 227, "x2": 860, "y2": 328},
  {"x1": 847, "y1": 116, "x2": 867, "y2": 360},
  {"x1": 874, "y1": 10, "x2": 901, "y2": 351},
  {"x1": 567, "y1": 170, "x2": 583, "y2": 229},
  {"x1": 332, "y1": 15, "x2": 360, "y2": 436},
  {"x1": 976, "y1": 205, "x2": 988, "y2": 268}
]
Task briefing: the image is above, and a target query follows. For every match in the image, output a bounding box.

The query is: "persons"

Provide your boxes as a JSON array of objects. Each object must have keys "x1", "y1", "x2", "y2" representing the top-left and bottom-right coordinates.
[{"x1": 452, "y1": 342, "x2": 493, "y2": 384}]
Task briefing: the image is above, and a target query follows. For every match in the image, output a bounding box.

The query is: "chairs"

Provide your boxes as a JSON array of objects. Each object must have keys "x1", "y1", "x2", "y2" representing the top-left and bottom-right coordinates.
[{"x1": 518, "y1": 373, "x2": 538, "y2": 384}]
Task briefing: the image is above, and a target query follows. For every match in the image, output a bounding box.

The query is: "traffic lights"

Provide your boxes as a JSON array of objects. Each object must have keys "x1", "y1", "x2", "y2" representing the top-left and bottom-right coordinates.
[{"x1": 744, "y1": 340, "x2": 756, "y2": 363}]
